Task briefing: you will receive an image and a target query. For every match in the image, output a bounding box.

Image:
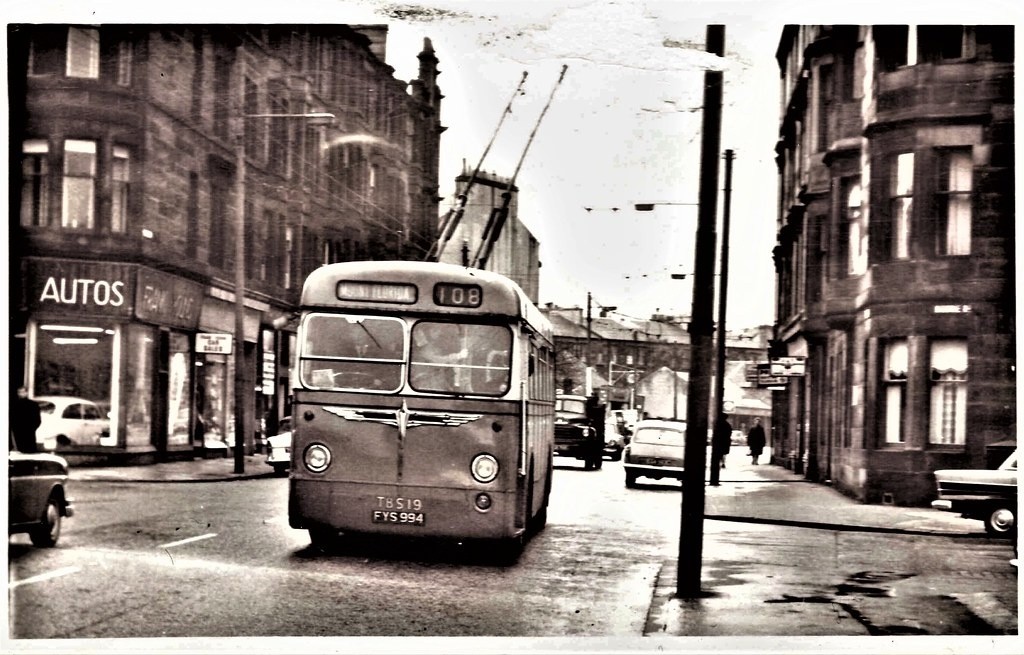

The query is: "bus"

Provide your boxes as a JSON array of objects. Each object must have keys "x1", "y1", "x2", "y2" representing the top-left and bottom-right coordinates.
[{"x1": 277, "y1": 261, "x2": 558, "y2": 548}]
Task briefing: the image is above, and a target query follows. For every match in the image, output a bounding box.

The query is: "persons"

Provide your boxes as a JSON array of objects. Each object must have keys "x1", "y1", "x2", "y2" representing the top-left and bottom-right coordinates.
[
  {"x1": 747, "y1": 417, "x2": 766, "y2": 465},
  {"x1": 719, "y1": 413, "x2": 732, "y2": 468}
]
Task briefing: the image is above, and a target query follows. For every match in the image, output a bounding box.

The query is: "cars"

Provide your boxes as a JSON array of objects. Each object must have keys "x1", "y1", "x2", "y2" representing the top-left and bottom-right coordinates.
[
  {"x1": 9, "y1": 440, "x2": 73, "y2": 547},
  {"x1": 33, "y1": 396, "x2": 110, "y2": 448},
  {"x1": 604, "y1": 423, "x2": 624, "y2": 462},
  {"x1": 623, "y1": 421, "x2": 685, "y2": 486},
  {"x1": 266, "y1": 416, "x2": 292, "y2": 473},
  {"x1": 933, "y1": 444, "x2": 1016, "y2": 541}
]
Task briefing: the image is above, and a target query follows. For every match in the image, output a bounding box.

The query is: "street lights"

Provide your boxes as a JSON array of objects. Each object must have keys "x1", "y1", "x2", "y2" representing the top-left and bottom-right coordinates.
[
  {"x1": 672, "y1": 272, "x2": 728, "y2": 485},
  {"x1": 234, "y1": 111, "x2": 336, "y2": 473},
  {"x1": 636, "y1": 201, "x2": 719, "y2": 598}
]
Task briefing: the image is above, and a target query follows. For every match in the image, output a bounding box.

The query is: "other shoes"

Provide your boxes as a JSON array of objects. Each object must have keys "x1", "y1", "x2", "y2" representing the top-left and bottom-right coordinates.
[
  {"x1": 721, "y1": 464, "x2": 726, "y2": 468},
  {"x1": 752, "y1": 462, "x2": 758, "y2": 465}
]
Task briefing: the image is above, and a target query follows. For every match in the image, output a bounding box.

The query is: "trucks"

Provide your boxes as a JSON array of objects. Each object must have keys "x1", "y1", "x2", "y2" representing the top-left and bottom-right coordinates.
[{"x1": 551, "y1": 395, "x2": 605, "y2": 468}]
